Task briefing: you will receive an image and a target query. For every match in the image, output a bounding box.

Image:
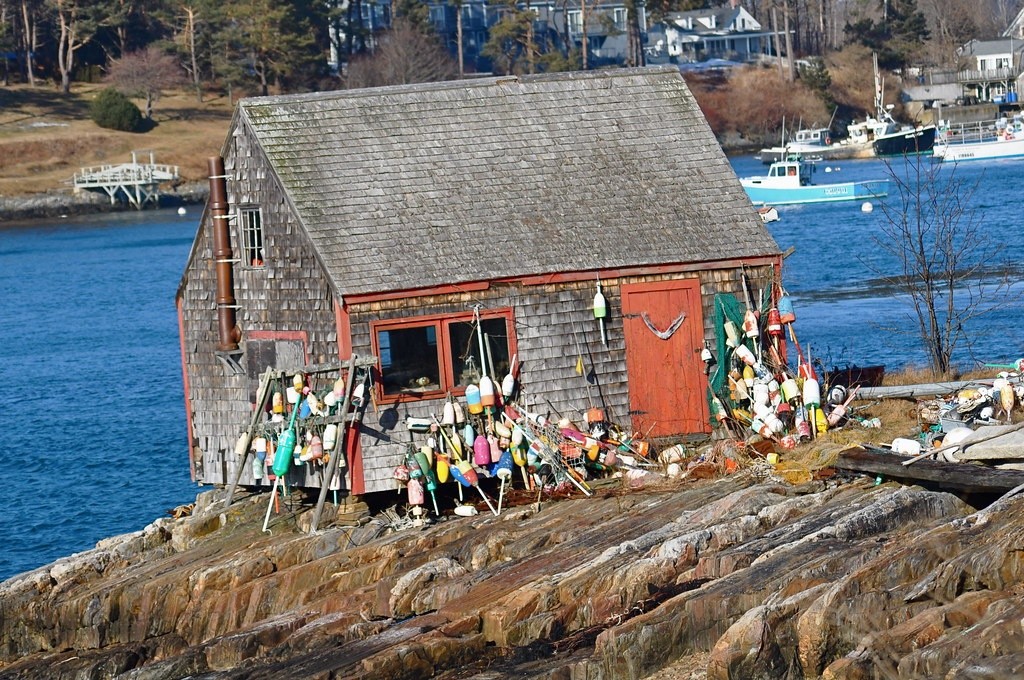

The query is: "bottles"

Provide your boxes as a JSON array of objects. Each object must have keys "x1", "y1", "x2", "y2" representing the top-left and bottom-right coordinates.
[{"x1": 415, "y1": 377, "x2": 430, "y2": 386}]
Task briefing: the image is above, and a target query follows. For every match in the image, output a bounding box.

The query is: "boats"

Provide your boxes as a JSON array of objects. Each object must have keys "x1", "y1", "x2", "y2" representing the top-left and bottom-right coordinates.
[
  {"x1": 760, "y1": 102, "x2": 879, "y2": 166},
  {"x1": 739, "y1": 145, "x2": 890, "y2": 207},
  {"x1": 829, "y1": 76, "x2": 937, "y2": 158},
  {"x1": 927, "y1": 104, "x2": 1024, "y2": 163}
]
